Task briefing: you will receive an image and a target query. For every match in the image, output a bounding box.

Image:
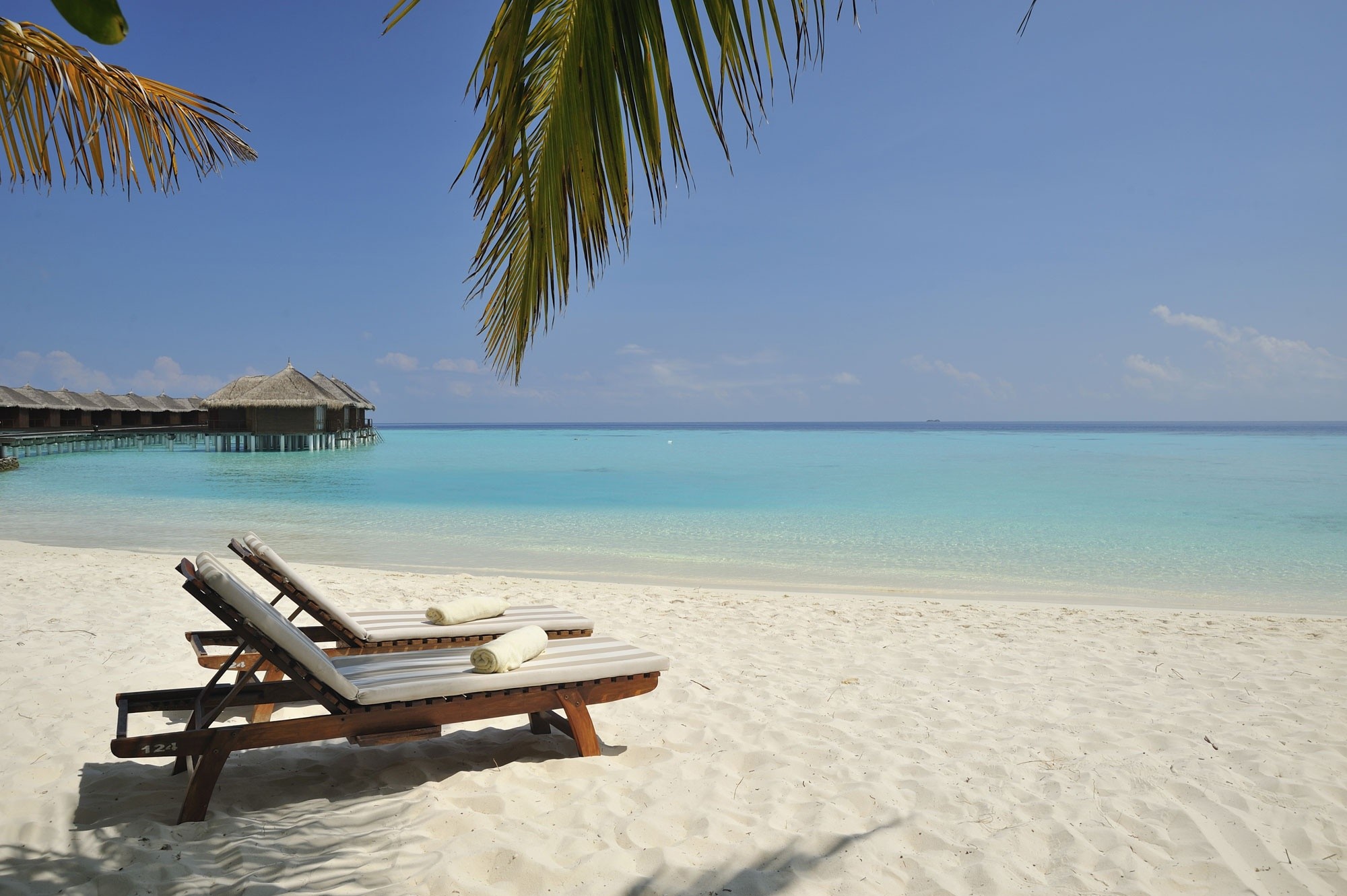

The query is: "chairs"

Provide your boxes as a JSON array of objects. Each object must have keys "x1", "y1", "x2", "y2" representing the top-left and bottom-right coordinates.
[{"x1": 111, "y1": 531, "x2": 671, "y2": 825}]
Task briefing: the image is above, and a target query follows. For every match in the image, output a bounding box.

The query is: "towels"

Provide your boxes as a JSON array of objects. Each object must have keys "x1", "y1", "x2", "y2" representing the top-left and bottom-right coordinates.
[
  {"x1": 470, "y1": 624, "x2": 549, "y2": 675},
  {"x1": 425, "y1": 597, "x2": 512, "y2": 625}
]
